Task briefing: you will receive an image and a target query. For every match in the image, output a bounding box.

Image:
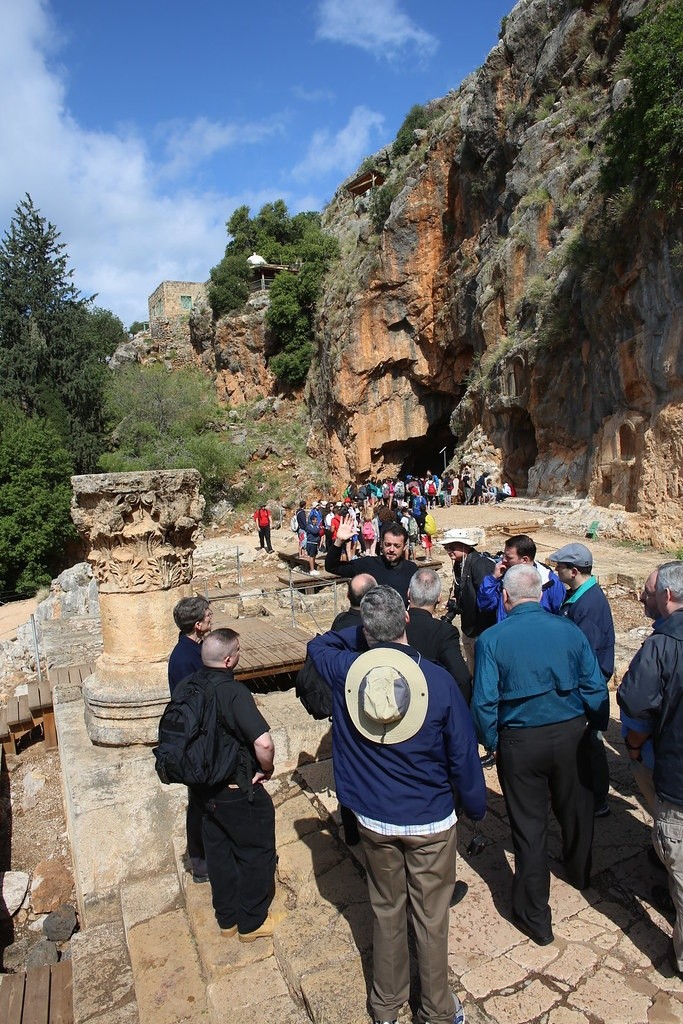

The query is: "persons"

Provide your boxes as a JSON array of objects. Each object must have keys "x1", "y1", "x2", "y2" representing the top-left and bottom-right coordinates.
[
  {"x1": 345, "y1": 476, "x2": 395, "y2": 510},
  {"x1": 395, "y1": 469, "x2": 452, "y2": 509},
  {"x1": 405, "y1": 568, "x2": 469, "y2": 908},
  {"x1": 330, "y1": 574, "x2": 378, "y2": 847},
  {"x1": 617, "y1": 561, "x2": 683, "y2": 976},
  {"x1": 295, "y1": 499, "x2": 382, "y2": 575},
  {"x1": 256, "y1": 502, "x2": 275, "y2": 554},
  {"x1": 549, "y1": 542, "x2": 615, "y2": 817},
  {"x1": 170, "y1": 628, "x2": 287, "y2": 943},
  {"x1": 168, "y1": 598, "x2": 212, "y2": 883},
  {"x1": 436, "y1": 529, "x2": 498, "y2": 686},
  {"x1": 378, "y1": 486, "x2": 432, "y2": 564},
  {"x1": 469, "y1": 563, "x2": 610, "y2": 946},
  {"x1": 324, "y1": 513, "x2": 418, "y2": 610},
  {"x1": 477, "y1": 535, "x2": 565, "y2": 623},
  {"x1": 449, "y1": 467, "x2": 497, "y2": 505},
  {"x1": 496, "y1": 480, "x2": 511, "y2": 503},
  {"x1": 307, "y1": 585, "x2": 489, "y2": 1024}
]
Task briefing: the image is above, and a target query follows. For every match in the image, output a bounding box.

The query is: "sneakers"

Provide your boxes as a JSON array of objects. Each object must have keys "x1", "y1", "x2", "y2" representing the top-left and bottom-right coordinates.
[
  {"x1": 450, "y1": 993, "x2": 464, "y2": 1024},
  {"x1": 238, "y1": 908, "x2": 287, "y2": 943},
  {"x1": 219, "y1": 924, "x2": 237, "y2": 938}
]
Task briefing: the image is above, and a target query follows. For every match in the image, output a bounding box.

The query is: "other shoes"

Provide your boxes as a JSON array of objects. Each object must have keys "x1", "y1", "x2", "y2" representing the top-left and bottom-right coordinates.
[
  {"x1": 268, "y1": 550, "x2": 275, "y2": 554},
  {"x1": 511, "y1": 907, "x2": 554, "y2": 945},
  {"x1": 310, "y1": 570, "x2": 321, "y2": 576},
  {"x1": 430, "y1": 507, "x2": 433, "y2": 510},
  {"x1": 595, "y1": 797, "x2": 610, "y2": 817},
  {"x1": 479, "y1": 752, "x2": 496, "y2": 768},
  {"x1": 450, "y1": 880, "x2": 468, "y2": 906},
  {"x1": 420, "y1": 560, "x2": 430, "y2": 564}
]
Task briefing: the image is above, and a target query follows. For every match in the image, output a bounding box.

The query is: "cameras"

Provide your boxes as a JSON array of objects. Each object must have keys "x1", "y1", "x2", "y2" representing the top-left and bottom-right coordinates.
[
  {"x1": 441, "y1": 599, "x2": 464, "y2": 623},
  {"x1": 467, "y1": 838, "x2": 488, "y2": 856}
]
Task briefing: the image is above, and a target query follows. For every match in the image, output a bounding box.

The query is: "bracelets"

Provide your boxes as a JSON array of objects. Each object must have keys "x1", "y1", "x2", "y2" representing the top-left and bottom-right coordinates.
[{"x1": 624, "y1": 738, "x2": 641, "y2": 750}]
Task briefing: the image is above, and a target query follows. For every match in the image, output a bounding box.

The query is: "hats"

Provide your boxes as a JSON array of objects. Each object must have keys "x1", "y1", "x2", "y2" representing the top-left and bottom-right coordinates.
[
  {"x1": 260, "y1": 504, "x2": 265, "y2": 507},
  {"x1": 343, "y1": 498, "x2": 351, "y2": 504},
  {"x1": 549, "y1": 543, "x2": 592, "y2": 567},
  {"x1": 437, "y1": 529, "x2": 478, "y2": 547},
  {"x1": 311, "y1": 501, "x2": 319, "y2": 509},
  {"x1": 343, "y1": 647, "x2": 430, "y2": 743}
]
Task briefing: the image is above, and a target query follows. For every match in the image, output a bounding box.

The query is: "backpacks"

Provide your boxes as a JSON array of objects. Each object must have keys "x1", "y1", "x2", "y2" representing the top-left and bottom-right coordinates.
[
  {"x1": 298, "y1": 528, "x2": 308, "y2": 550},
  {"x1": 153, "y1": 670, "x2": 245, "y2": 788},
  {"x1": 381, "y1": 484, "x2": 406, "y2": 498},
  {"x1": 290, "y1": 510, "x2": 302, "y2": 532},
  {"x1": 362, "y1": 521, "x2": 375, "y2": 539},
  {"x1": 427, "y1": 482, "x2": 436, "y2": 495},
  {"x1": 424, "y1": 514, "x2": 437, "y2": 534},
  {"x1": 404, "y1": 514, "x2": 418, "y2": 541}
]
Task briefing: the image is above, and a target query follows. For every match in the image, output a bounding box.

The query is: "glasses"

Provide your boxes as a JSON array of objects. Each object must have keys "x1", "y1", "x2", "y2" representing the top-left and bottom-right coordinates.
[{"x1": 443, "y1": 543, "x2": 460, "y2": 551}]
